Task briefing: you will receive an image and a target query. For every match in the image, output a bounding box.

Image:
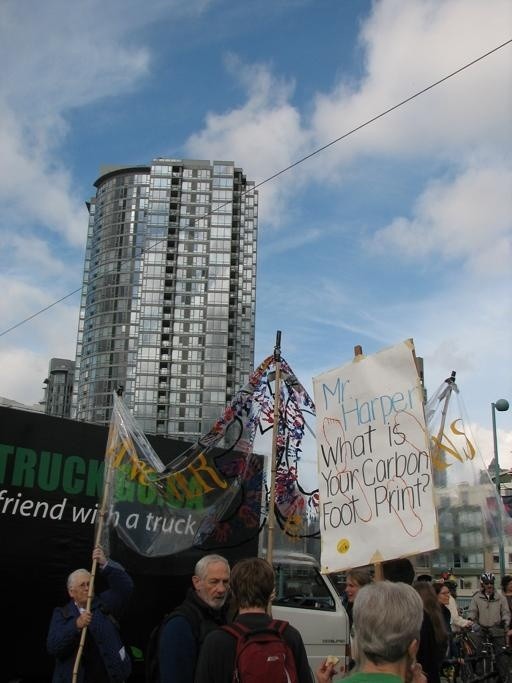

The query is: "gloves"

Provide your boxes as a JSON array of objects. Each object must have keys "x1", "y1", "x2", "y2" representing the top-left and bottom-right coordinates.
[{"x1": 471, "y1": 623, "x2": 481, "y2": 632}]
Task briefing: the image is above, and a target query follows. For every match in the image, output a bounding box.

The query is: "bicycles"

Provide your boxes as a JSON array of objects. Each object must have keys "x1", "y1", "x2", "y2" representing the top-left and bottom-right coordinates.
[{"x1": 442, "y1": 621, "x2": 512, "y2": 682}]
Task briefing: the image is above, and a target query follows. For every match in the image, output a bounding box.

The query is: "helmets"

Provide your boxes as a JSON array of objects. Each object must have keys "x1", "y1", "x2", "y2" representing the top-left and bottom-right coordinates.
[{"x1": 481, "y1": 573, "x2": 495, "y2": 584}]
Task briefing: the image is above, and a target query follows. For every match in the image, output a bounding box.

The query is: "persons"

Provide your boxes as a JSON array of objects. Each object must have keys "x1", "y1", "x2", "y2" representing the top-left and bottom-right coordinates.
[
  {"x1": 344, "y1": 559, "x2": 511, "y2": 682},
  {"x1": 45, "y1": 549, "x2": 134, "y2": 683},
  {"x1": 315, "y1": 580, "x2": 429, "y2": 683},
  {"x1": 158, "y1": 552, "x2": 231, "y2": 683},
  {"x1": 195, "y1": 556, "x2": 314, "y2": 683}
]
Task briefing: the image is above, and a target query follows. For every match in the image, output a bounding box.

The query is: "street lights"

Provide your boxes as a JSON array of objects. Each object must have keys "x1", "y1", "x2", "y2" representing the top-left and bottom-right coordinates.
[{"x1": 487, "y1": 397, "x2": 509, "y2": 587}]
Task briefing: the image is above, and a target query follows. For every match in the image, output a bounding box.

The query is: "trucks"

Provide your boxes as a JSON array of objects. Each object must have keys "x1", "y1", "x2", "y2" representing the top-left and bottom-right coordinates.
[{"x1": 1, "y1": 405, "x2": 353, "y2": 683}]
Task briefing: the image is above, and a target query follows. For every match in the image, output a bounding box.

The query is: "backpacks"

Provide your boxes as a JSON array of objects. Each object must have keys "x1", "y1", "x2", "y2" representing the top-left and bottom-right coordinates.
[{"x1": 218, "y1": 619, "x2": 299, "y2": 683}]
